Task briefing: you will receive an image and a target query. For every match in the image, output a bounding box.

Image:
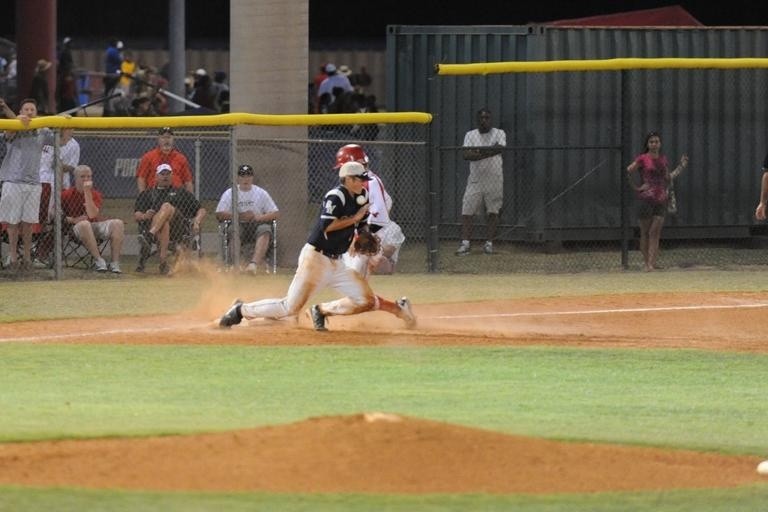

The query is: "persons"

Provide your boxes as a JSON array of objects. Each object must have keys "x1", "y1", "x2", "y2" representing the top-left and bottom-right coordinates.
[
  {"x1": 216, "y1": 164, "x2": 279, "y2": 272},
  {"x1": 217, "y1": 160, "x2": 380, "y2": 332},
  {"x1": 456, "y1": 107, "x2": 507, "y2": 257},
  {"x1": 1, "y1": 37, "x2": 230, "y2": 117},
  {"x1": 755, "y1": 159, "x2": 767, "y2": 224},
  {"x1": 628, "y1": 133, "x2": 689, "y2": 272},
  {"x1": 0, "y1": 98, "x2": 123, "y2": 272},
  {"x1": 308, "y1": 64, "x2": 380, "y2": 140},
  {"x1": 333, "y1": 143, "x2": 414, "y2": 327},
  {"x1": 133, "y1": 127, "x2": 206, "y2": 273}
]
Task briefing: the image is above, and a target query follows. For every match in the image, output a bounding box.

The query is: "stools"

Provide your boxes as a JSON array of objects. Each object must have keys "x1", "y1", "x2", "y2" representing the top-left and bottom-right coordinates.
[
  {"x1": 0, "y1": 218, "x2": 60, "y2": 274},
  {"x1": 215, "y1": 211, "x2": 278, "y2": 277},
  {"x1": 39, "y1": 218, "x2": 114, "y2": 270},
  {"x1": 133, "y1": 213, "x2": 203, "y2": 279}
]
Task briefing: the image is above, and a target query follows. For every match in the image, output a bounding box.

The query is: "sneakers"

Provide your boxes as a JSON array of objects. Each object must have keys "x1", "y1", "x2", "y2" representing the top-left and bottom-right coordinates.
[
  {"x1": 306, "y1": 305, "x2": 328, "y2": 331},
  {"x1": 109, "y1": 261, "x2": 123, "y2": 273},
  {"x1": 138, "y1": 232, "x2": 153, "y2": 247},
  {"x1": 244, "y1": 261, "x2": 257, "y2": 276},
  {"x1": 483, "y1": 241, "x2": 494, "y2": 255},
  {"x1": 93, "y1": 258, "x2": 109, "y2": 271},
  {"x1": 217, "y1": 297, "x2": 244, "y2": 328},
  {"x1": 158, "y1": 260, "x2": 171, "y2": 275},
  {"x1": 454, "y1": 240, "x2": 472, "y2": 257},
  {"x1": 230, "y1": 264, "x2": 244, "y2": 275},
  {"x1": 395, "y1": 297, "x2": 416, "y2": 330}
]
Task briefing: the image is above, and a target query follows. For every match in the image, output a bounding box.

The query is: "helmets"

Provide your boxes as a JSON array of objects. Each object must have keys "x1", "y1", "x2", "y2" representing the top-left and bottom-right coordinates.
[{"x1": 332, "y1": 143, "x2": 371, "y2": 168}]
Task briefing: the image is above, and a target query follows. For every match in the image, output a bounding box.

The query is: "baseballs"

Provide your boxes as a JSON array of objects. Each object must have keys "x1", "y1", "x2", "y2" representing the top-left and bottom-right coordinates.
[{"x1": 356, "y1": 195, "x2": 366, "y2": 206}]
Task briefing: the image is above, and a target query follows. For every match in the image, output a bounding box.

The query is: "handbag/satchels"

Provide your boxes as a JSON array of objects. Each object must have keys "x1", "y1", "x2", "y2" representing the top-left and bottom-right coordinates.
[{"x1": 664, "y1": 174, "x2": 680, "y2": 215}]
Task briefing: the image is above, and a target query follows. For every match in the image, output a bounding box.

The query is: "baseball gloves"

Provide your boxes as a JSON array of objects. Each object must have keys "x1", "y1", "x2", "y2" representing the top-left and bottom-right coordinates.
[{"x1": 355, "y1": 230, "x2": 381, "y2": 255}]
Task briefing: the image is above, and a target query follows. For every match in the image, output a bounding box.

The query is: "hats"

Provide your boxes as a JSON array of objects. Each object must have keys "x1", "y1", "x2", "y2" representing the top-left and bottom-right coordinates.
[
  {"x1": 214, "y1": 70, "x2": 226, "y2": 79},
  {"x1": 34, "y1": 59, "x2": 53, "y2": 73},
  {"x1": 325, "y1": 63, "x2": 336, "y2": 73},
  {"x1": 238, "y1": 165, "x2": 255, "y2": 177},
  {"x1": 336, "y1": 64, "x2": 352, "y2": 76},
  {"x1": 156, "y1": 164, "x2": 173, "y2": 175},
  {"x1": 338, "y1": 162, "x2": 373, "y2": 182},
  {"x1": 159, "y1": 125, "x2": 174, "y2": 136}
]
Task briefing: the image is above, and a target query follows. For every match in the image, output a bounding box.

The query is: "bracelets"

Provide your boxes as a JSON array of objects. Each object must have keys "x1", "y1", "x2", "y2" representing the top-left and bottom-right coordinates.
[
  {"x1": 680, "y1": 161, "x2": 685, "y2": 171},
  {"x1": 475, "y1": 148, "x2": 480, "y2": 153}
]
